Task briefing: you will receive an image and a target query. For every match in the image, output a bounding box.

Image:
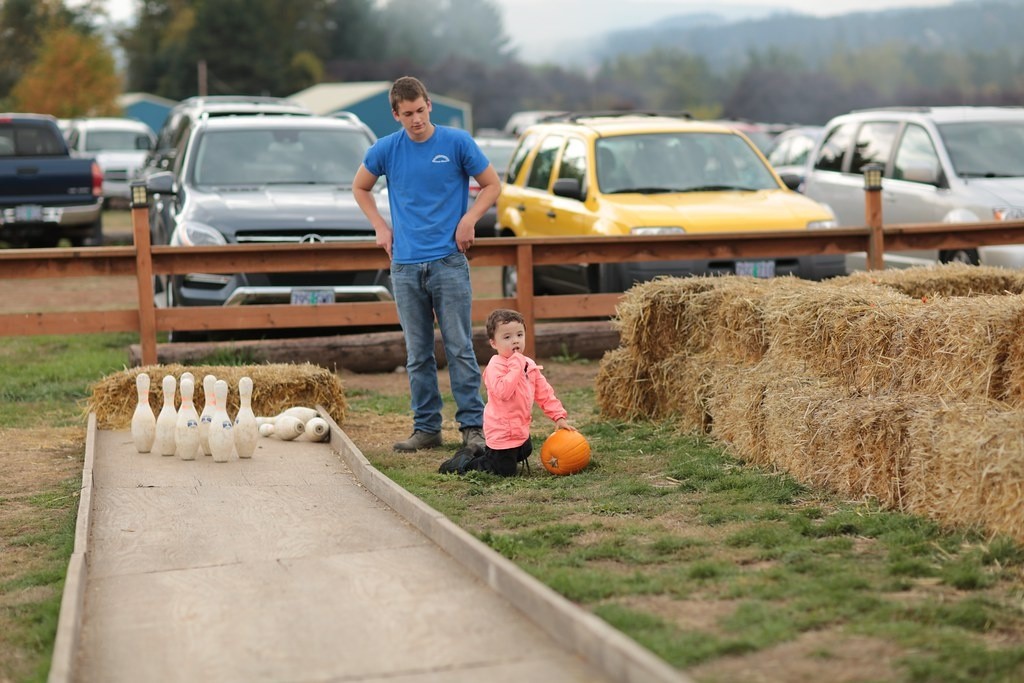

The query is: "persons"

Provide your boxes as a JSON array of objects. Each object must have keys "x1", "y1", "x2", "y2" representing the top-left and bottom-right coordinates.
[
  {"x1": 439, "y1": 309, "x2": 579, "y2": 477},
  {"x1": 352, "y1": 77, "x2": 502, "y2": 452}
]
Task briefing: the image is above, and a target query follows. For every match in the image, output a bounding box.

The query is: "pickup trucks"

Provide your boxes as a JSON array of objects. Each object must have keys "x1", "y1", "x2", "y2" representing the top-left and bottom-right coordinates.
[{"x1": 0, "y1": 113, "x2": 105, "y2": 249}]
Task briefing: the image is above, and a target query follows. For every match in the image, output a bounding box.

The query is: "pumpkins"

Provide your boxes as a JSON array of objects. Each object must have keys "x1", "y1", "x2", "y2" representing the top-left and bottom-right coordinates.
[{"x1": 540, "y1": 428, "x2": 590, "y2": 475}]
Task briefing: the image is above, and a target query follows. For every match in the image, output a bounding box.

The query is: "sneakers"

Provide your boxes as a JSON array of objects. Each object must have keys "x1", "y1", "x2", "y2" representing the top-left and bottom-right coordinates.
[
  {"x1": 393, "y1": 429, "x2": 442, "y2": 453},
  {"x1": 439, "y1": 449, "x2": 475, "y2": 473},
  {"x1": 461, "y1": 427, "x2": 485, "y2": 448}
]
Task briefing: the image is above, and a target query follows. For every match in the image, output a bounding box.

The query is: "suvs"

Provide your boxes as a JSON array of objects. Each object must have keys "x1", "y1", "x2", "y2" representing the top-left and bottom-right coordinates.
[
  {"x1": 493, "y1": 111, "x2": 847, "y2": 300},
  {"x1": 137, "y1": 97, "x2": 317, "y2": 233},
  {"x1": 137, "y1": 110, "x2": 437, "y2": 343}
]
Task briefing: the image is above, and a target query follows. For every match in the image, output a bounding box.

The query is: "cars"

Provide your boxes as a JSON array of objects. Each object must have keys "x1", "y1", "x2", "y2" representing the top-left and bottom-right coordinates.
[
  {"x1": 64, "y1": 114, "x2": 159, "y2": 211},
  {"x1": 467, "y1": 110, "x2": 567, "y2": 235},
  {"x1": 729, "y1": 126, "x2": 822, "y2": 194}
]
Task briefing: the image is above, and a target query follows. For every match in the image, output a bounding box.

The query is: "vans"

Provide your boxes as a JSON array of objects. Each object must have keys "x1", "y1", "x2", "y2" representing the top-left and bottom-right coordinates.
[{"x1": 805, "y1": 107, "x2": 1024, "y2": 275}]
